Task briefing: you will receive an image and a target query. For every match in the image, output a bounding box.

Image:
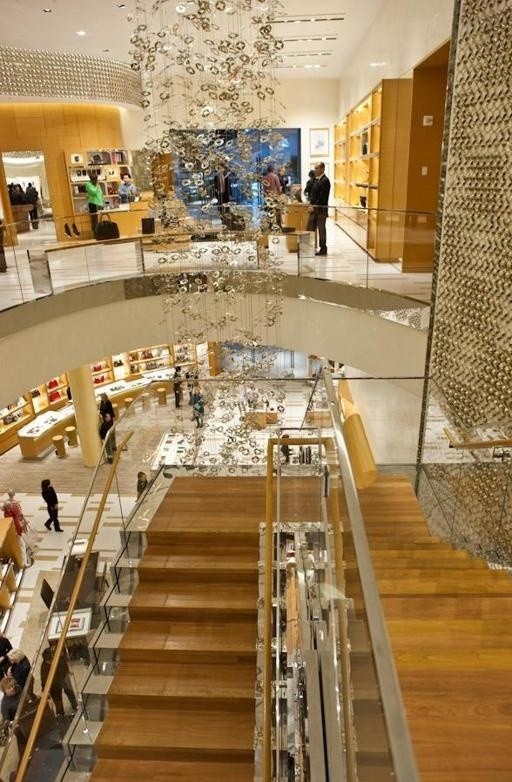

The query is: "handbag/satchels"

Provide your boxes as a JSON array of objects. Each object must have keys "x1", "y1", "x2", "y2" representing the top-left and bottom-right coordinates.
[{"x1": 94, "y1": 213, "x2": 119, "y2": 240}]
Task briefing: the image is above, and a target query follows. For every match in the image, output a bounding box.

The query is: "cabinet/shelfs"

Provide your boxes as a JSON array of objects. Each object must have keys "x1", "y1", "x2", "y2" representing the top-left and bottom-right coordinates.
[
  {"x1": 63, "y1": 149, "x2": 141, "y2": 215},
  {"x1": 0, "y1": 517, "x2": 31, "y2": 633},
  {"x1": 334, "y1": 79, "x2": 413, "y2": 263},
  {"x1": 0, "y1": 344, "x2": 215, "y2": 458}
]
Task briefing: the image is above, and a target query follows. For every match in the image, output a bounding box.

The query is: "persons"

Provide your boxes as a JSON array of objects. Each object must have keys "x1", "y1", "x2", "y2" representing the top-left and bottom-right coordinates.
[
  {"x1": 303, "y1": 170, "x2": 318, "y2": 231},
  {"x1": 25, "y1": 183, "x2": 40, "y2": 229},
  {"x1": 12, "y1": 185, "x2": 23, "y2": 194},
  {"x1": 213, "y1": 166, "x2": 229, "y2": 211},
  {"x1": 262, "y1": 165, "x2": 282, "y2": 232},
  {"x1": 172, "y1": 365, "x2": 200, "y2": 408},
  {"x1": 136, "y1": 472, "x2": 147, "y2": 497},
  {"x1": 307, "y1": 161, "x2": 331, "y2": 256},
  {"x1": 100, "y1": 412, "x2": 114, "y2": 464},
  {"x1": 118, "y1": 172, "x2": 138, "y2": 201},
  {"x1": 188, "y1": 386, "x2": 206, "y2": 429},
  {"x1": 279, "y1": 434, "x2": 296, "y2": 464},
  {"x1": 0, "y1": 502, "x2": 32, "y2": 568},
  {"x1": 1, "y1": 675, "x2": 24, "y2": 722},
  {"x1": 40, "y1": 478, "x2": 65, "y2": 532},
  {"x1": 5, "y1": 488, "x2": 28, "y2": 534},
  {"x1": 85, "y1": 173, "x2": 105, "y2": 236},
  {"x1": 6, "y1": 648, "x2": 38, "y2": 703},
  {"x1": 99, "y1": 393, "x2": 118, "y2": 452},
  {"x1": 277, "y1": 165, "x2": 292, "y2": 193},
  {"x1": 79, "y1": 627, "x2": 97, "y2": 670},
  {"x1": 40, "y1": 644, "x2": 81, "y2": 718},
  {"x1": 1, "y1": 630, "x2": 13, "y2": 682}
]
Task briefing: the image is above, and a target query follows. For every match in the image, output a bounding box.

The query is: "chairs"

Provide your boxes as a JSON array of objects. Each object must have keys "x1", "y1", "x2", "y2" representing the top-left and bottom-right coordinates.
[{"x1": 41, "y1": 537, "x2": 111, "y2": 663}]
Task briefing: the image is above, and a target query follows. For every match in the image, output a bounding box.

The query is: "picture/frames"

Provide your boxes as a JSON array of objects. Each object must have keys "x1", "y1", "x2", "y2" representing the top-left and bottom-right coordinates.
[
  {"x1": 309, "y1": 128, "x2": 330, "y2": 158},
  {"x1": 309, "y1": 161, "x2": 330, "y2": 181}
]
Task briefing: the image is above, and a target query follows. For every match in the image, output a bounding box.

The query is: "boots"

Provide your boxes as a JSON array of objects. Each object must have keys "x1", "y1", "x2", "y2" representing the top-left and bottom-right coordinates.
[{"x1": 315, "y1": 247, "x2": 326, "y2": 255}]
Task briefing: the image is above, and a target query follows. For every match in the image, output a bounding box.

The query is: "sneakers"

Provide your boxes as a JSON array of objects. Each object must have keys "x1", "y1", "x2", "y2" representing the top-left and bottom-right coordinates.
[
  {"x1": 55, "y1": 528, "x2": 64, "y2": 532},
  {"x1": 45, "y1": 522, "x2": 52, "y2": 531}
]
